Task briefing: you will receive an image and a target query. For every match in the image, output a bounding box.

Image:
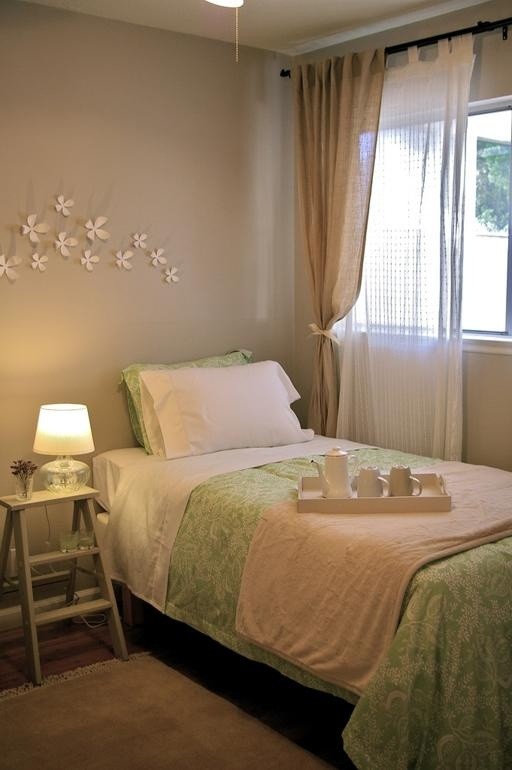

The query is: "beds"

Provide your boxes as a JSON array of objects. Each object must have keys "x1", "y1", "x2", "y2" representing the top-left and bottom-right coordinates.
[{"x1": 92, "y1": 430, "x2": 511, "y2": 770}]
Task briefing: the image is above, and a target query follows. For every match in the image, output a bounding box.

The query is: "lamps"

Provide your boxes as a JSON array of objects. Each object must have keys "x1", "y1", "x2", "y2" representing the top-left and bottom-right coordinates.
[{"x1": 31, "y1": 403, "x2": 93, "y2": 495}]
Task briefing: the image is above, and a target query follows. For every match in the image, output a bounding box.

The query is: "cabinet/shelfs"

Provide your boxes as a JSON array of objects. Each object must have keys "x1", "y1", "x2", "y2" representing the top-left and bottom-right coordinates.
[{"x1": 1, "y1": 486, "x2": 127, "y2": 685}]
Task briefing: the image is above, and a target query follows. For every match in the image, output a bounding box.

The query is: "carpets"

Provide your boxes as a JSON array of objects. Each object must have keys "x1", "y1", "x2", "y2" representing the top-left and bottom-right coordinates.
[{"x1": 0, "y1": 650, "x2": 355, "y2": 769}]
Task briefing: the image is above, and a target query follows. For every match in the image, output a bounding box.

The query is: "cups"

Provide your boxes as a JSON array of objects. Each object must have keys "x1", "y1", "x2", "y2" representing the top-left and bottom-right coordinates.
[
  {"x1": 390, "y1": 463, "x2": 422, "y2": 496},
  {"x1": 59, "y1": 528, "x2": 95, "y2": 553},
  {"x1": 357, "y1": 464, "x2": 391, "y2": 497}
]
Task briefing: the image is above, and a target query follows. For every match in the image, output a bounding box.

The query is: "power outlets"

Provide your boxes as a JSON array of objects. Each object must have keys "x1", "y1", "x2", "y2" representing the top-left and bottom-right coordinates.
[{"x1": 8, "y1": 546, "x2": 24, "y2": 579}]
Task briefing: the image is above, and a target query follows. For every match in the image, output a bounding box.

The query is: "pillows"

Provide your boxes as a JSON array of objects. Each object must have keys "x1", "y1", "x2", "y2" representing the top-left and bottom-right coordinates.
[
  {"x1": 118, "y1": 349, "x2": 257, "y2": 450},
  {"x1": 140, "y1": 360, "x2": 312, "y2": 452}
]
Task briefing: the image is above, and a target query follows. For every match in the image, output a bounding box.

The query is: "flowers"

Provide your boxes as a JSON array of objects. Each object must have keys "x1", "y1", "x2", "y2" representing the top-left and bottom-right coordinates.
[{"x1": 9, "y1": 459, "x2": 38, "y2": 478}]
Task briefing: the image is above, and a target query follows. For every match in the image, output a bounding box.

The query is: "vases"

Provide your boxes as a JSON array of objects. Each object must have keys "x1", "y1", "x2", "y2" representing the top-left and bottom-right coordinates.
[{"x1": 18, "y1": 478, "x2": 28, "y2": 501}]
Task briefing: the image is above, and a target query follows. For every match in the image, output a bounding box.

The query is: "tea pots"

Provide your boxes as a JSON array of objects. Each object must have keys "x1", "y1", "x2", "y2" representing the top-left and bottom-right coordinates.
[{"x1": 311, "y1": 446, "x2": 358, "y2": 498}]
